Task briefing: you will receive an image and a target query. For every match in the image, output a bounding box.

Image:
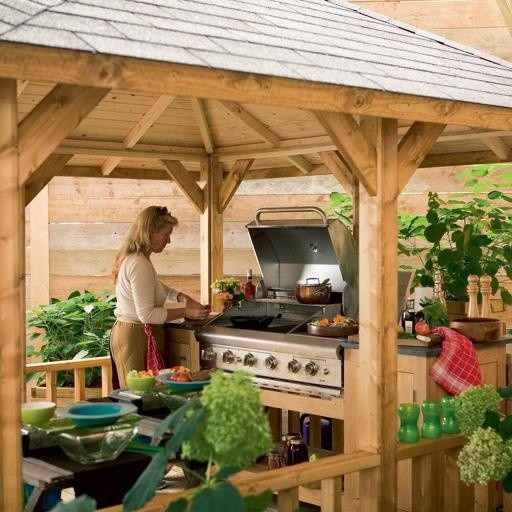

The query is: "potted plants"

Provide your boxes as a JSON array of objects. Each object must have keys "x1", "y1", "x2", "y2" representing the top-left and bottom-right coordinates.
[{"x1": 323, "y1": 162, "x2": 512, "y2": 322}]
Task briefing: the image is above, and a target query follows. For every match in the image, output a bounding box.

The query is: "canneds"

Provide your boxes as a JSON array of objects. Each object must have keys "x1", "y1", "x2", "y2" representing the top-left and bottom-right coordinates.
[{"x1": 269, "y1": 433, "x2": 308, "y2": 469}]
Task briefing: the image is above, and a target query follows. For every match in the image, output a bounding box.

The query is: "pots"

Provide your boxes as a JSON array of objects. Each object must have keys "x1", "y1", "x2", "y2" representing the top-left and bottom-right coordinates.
[
  {"x1": 227, "y1": 314, "x2": 282, "y2": 331},
  {"x1": 295, "y1": 276, "x2": 334, "y2": 304}
]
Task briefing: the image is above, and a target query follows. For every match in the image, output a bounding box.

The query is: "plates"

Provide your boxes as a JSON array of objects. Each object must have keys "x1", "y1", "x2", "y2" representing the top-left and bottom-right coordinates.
[
  {"x1": 34, "y1": 410, "x2": 143, "y2": 431},
  {"x1": 155, "y1": 373, "x2": 212, "y2": 390},
  {"x1": 55, "y1": 401, "x2": 139, "y2": 428}
]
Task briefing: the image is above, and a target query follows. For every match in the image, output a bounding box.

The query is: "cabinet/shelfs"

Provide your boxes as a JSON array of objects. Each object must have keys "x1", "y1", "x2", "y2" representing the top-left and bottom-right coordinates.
[
  {"x1": 341, "y1": 345, "x2": 506, "y2": 510},
  {"x1": 161, "y1": 325, "x2": 201, "y2": 373}
]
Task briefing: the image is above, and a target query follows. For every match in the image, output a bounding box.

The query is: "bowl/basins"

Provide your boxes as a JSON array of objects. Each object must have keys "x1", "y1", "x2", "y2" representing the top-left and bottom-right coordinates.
[
  {"x1": 307, "y1": 323, "x2": 351, "y2": 336},
  {"x1": 449, "y1": 318, "x2": 506, "y2": 342},
  {"x1": 158, "y1": 369, "x2": 171, "y2": 379},
  {"x1": 125, "y1": 377, "x2": 155, "y2": 394},
  {"x1": 49, "y1": 425, "x2": 138, "y2": 464},
  {"x1": 22, "y1": 402, "x2": 57, "y2": 423}
]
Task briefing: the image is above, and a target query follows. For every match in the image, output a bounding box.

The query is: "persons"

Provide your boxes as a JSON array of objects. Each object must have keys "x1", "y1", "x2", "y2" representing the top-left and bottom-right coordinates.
[{"x1": 108, "y1": 206, "x2": 210, "y2": 390}]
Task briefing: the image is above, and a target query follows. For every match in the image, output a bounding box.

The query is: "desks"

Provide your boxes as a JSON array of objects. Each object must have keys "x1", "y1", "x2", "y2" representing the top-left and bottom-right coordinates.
[{"x1": 21, "y1": 444, "x2": 152, "y2": 512}]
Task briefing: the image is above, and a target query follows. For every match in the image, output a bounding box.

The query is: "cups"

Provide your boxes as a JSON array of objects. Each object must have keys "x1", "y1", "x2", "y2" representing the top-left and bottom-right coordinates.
[{"x1": 398, "y1": 395, "x2": 462, "y2": 442}]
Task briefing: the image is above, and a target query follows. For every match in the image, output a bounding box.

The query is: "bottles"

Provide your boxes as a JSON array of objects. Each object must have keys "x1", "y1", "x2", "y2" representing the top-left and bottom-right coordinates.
[
  {"x1": 244, "y1": 269, "x2": 256, "y2": 299},
  {"x1": 267, "y1": 451, "x2": 284, "y2": 469},
  {"x1": 285, "y1": 433, "x2": 309, "y2": 465},
  {"x1": 402, "y1": 304, "x2": 416, "y2": 337}
]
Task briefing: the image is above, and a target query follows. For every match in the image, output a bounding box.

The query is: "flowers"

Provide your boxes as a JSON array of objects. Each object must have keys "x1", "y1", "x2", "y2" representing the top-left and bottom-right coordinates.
[{"x1": 209, "y1": 277, "x2": 246, "y2": 304}]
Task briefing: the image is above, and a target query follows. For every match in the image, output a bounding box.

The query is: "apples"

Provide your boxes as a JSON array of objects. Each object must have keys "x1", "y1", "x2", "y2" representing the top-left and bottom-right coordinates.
[{"x1": 309, "y1": 454, "x2": 318, "y2": 462}]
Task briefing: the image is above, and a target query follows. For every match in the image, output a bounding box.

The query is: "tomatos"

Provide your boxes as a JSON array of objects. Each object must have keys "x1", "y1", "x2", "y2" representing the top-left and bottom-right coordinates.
[{"x1": 170, "y1": 374, "x2": 190, "y2": 382}]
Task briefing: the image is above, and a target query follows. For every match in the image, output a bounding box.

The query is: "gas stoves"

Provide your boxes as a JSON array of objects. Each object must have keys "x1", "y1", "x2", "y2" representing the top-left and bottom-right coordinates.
[{"x1": 194, "y1": 318, "x2": 348, "y2": 399}]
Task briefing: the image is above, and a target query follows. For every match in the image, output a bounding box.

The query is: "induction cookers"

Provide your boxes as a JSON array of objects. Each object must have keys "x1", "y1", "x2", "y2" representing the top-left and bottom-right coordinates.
[{"x1": 209, "y1": 283, "x2": 341, "y2": 334}]
